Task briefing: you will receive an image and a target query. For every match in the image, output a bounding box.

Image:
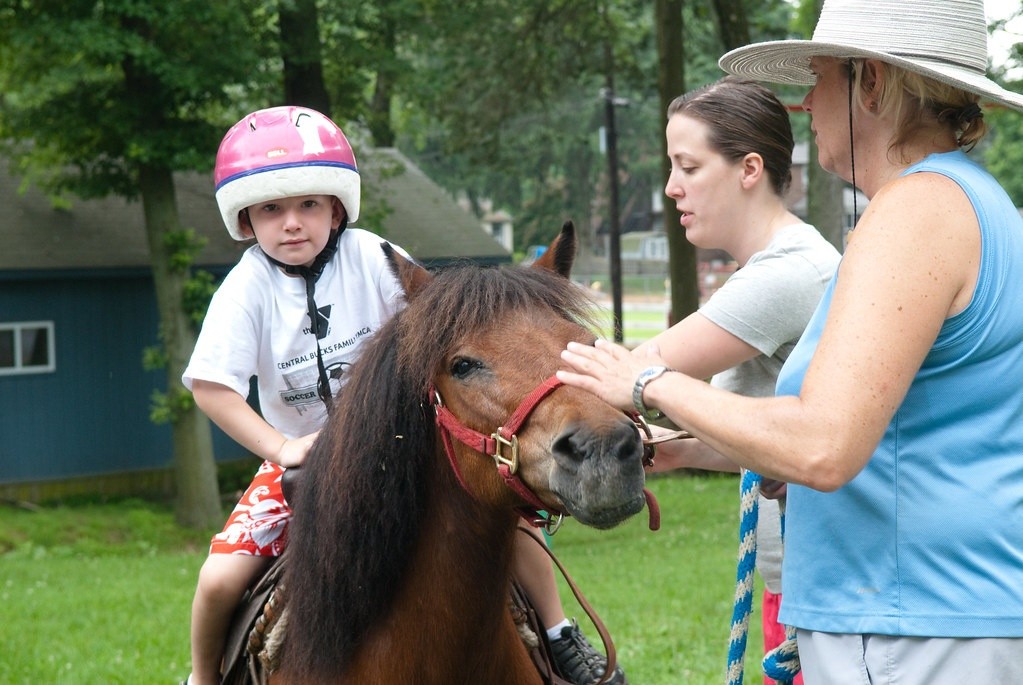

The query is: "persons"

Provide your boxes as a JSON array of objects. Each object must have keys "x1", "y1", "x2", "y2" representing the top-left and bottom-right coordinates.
[
  {"x1": 181, "y1": 106, "x2": 627, "y2": 685},
  {"x1": 631, "y1": 75, "x2": 848, "y2": 685},
  {"x1": 556, "y1": 0, "x2": 1023, "y2": 685}
]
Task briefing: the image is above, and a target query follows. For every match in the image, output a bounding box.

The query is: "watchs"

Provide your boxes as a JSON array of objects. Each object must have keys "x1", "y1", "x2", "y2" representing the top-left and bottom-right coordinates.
[{"x1": 633, "y1": 366, "x2": 680, "y2": 422}]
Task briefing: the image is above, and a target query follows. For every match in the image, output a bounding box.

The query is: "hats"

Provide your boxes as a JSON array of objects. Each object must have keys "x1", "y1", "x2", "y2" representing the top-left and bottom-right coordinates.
[{"x1": 718, "y1": 0, "x2": 1023, "y2": 114}]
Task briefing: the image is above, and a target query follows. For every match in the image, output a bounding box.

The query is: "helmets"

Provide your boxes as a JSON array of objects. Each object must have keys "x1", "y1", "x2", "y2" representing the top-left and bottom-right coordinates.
[{"x1": 213, "y1": 106, "x2": 361, "y2": 242}]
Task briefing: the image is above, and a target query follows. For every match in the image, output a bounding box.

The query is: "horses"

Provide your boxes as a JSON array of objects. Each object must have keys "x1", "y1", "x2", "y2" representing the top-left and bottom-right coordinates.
[{"x1": 216, "y1": 220, "x2": 649, "y2": 684}]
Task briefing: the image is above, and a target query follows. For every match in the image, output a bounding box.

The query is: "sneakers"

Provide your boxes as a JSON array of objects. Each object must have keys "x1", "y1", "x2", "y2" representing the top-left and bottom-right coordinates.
[{"x1": 549, "y1": 619, "x2": 624, "y2": 685}]
